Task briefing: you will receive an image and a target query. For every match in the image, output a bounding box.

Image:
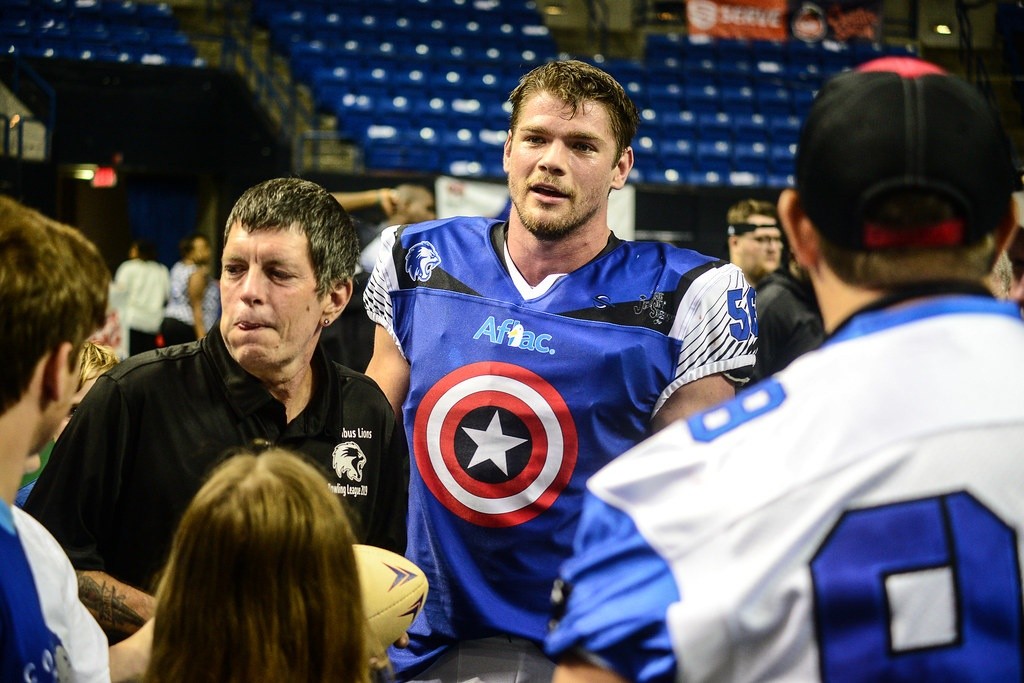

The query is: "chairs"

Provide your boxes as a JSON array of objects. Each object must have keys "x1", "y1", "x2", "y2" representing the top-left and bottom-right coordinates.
[{"x1": 0, "y1": 1, "x2": 920, "y2": 198}]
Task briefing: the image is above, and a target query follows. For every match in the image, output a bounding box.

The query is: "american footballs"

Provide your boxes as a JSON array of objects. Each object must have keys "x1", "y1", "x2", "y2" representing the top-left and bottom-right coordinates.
[{"x1": 351, "y1": 544, "x2": 429, "y2": 661}]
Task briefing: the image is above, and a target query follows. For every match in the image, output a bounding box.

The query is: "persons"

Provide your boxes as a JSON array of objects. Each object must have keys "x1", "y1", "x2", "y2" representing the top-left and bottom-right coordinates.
[{"x1": 0, "y1": 56, "x2": 1024, "y2": 682}]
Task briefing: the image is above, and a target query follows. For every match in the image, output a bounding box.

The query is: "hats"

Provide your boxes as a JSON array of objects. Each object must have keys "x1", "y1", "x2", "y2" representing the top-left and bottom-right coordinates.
[{"x1": 796, "y1": 56, "x2": 1013, "y2": 251}]
